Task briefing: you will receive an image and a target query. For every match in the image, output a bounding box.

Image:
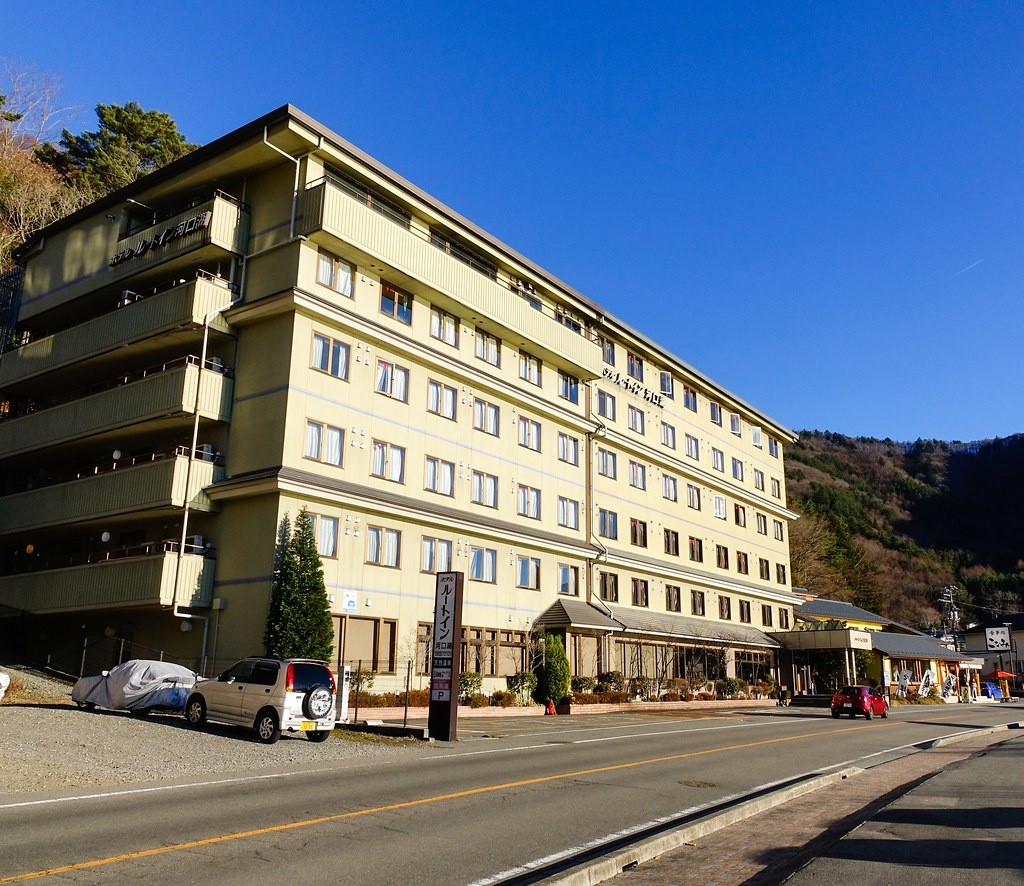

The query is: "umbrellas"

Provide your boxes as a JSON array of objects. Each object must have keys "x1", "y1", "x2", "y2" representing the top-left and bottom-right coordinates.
[{"x1": 984, "y1": 669, "x2": 1017, "y2": 689}]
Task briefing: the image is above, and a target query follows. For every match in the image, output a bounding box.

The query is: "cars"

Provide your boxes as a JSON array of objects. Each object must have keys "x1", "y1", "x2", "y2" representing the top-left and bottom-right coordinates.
[
  {"x1": 831, "y1": 685, "x2": 889, "y2": 719},
  {"x1": 979, "y1": 681, "x2": 1004, "y2": 700}
]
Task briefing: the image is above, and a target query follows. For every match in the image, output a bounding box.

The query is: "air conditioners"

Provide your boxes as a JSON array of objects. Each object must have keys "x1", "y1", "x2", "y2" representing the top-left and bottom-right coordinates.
[
  {"x1": 205, "y1": 357, "x2": 221, "y2": 373},
  {"x1": 154, "y1": 444, "x2": 213, "y2": 461},
  {"x1": 142, "y1": 535, "x2": 203, "y2": 554}
]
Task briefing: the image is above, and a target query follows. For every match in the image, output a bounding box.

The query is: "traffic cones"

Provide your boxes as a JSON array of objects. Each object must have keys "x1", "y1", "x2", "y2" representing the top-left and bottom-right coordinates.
[{"x1": 547, "y1": 696, "x2": 556, "y2": 715}]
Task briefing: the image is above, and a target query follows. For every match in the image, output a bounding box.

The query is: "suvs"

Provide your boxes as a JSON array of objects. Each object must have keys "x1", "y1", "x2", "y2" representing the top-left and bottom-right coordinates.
[{"x1": 183, "y1": 657, "x2": 338, "y2": 745}]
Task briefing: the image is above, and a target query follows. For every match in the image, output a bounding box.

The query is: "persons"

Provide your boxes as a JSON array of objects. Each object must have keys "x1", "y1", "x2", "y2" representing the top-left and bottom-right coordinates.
[{"x1": 971, "y1": 678, "x2": 978, "y2": 701}]
